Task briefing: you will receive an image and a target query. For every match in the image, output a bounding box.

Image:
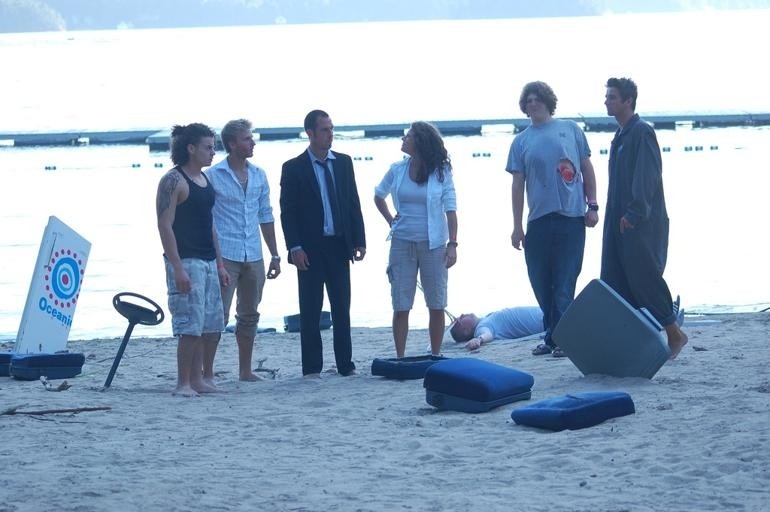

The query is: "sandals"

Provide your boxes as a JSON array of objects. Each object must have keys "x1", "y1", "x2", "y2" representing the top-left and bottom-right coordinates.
[
  {"x1": 550, "y1": 346, "x2": 567, "y2": 358},
  {"x1": 531, "y1": 342, "x2": 552, "y2": 356}
]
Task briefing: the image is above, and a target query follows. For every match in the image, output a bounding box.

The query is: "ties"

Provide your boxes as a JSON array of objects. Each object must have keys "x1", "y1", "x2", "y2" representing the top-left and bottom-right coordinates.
[{"x1": 315, "y1": 158, "x2": 346, "y2": 240}]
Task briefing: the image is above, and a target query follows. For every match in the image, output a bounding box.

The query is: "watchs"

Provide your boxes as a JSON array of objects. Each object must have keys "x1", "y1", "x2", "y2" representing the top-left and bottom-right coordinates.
[
  {"x1": 272, "y1": 256, "x2": 281, "y2": 262},
  {"x1": 589, "y1": 204, "x2": 598, "y2": 211},
  {"x1": 477, "y1": 336, "x2": 483, "y2": 344}
]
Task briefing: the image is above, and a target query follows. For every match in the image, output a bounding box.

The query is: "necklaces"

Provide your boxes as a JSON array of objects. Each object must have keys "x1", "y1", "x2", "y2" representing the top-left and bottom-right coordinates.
[
  {"x1": 231, "y1": 165, "x2": 247, "y2": 185},
  {"x1": 183, "y1": 168, "x2": 207, "y2": 187}
]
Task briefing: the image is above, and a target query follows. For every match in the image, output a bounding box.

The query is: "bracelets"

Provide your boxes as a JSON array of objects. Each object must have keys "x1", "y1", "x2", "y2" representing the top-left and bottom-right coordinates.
[
  {"x1": 384, "y1": 213, "x2": 391, "y2": 220},
  {"x1": 217, "y1": 264, "x2": 224, "y2": 268},
  {"x1": 588, "y1": 199, "x2": 597, "y2": 204},
  {"x1": 447, "y1": 241, "x2": 458, "y2": 247}
]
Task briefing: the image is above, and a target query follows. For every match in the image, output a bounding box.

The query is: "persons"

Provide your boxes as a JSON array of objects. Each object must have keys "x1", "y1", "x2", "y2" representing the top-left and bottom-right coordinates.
[
  {"x1": 450, "y1": 295, "x2": 684, "y2": 350},
  {"x1": 280, "y1": 110, "x2": 365, "y2": 377},
  {"x1": 156, "y1": 124, "x2": 231, "y2": 398},
  {"x1": 375, "y1": 120, "x2": 458, "y2": 359},
  {"x1": 505, "y1": 80, "x2": 600, "y2": 357},
  {"x1": 202, "y1": 119, "x2": 281, "y2": 389},
  {"x1": 600, "y1": 77, "x2": 688, "y2": 359}
]
